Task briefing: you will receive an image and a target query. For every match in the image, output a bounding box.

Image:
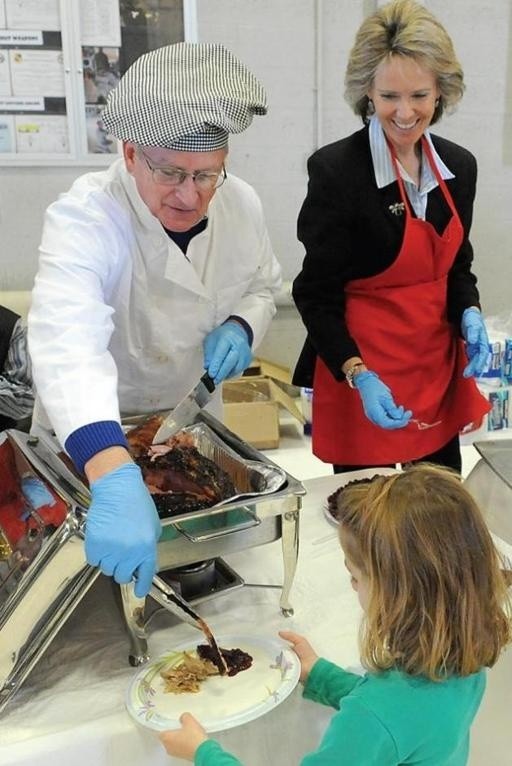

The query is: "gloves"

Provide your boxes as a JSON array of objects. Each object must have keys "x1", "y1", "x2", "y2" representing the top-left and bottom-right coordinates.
[
  {"x1": 197, "y1": 318, "x2": 257, "y2": 383},
  {"x1": 348, "y1": 374, "x2": 416, "y2": 434},
  {"x1": 457, "y1": 310, "x2": 491, "y2": 386},
  {"x1": 82, "y1": 463, "x2": 167, "y2": 603}
]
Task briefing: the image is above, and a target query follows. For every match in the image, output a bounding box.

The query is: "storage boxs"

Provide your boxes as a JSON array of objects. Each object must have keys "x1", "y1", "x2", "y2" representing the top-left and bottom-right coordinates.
[
  {"x1": 223, "y1": 379, "x2": 306, "y2": 447},
  {"x1": 233, "y1": 360, "x2": 294, "y2": 386}
]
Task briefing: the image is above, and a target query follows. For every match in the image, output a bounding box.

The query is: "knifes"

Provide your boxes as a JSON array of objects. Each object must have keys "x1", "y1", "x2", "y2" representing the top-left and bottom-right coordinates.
[{"x1": 152, "y1": 369, "x2": 217, "y2": 445}]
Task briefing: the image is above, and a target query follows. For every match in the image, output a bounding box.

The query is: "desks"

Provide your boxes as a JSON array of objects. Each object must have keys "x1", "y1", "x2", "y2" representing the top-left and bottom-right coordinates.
[{"x1": 2, "y1": 439, "x2": 512, "y2": 766}]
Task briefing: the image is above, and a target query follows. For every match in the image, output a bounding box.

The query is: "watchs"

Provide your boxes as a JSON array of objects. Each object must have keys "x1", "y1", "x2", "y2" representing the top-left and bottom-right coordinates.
[{"x1": 346, "y1": 362, "x2": 364, "y2": 389}]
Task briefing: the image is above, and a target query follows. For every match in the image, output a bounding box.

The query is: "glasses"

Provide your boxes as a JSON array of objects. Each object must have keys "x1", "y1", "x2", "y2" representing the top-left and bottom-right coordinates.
[{"x1": 135, "y1": 147, "x2": 228, "y2": 195}]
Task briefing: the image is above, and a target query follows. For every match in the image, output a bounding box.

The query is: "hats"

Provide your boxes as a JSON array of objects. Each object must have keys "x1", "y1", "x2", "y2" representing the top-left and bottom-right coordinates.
[{"x1": 98, "y1": 38, "x2": 273, "y2": 154}]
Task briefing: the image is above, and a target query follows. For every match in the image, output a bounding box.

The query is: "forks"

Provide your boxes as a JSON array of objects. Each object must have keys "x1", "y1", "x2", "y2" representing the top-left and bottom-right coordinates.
[{"x1": 393, "y1": 416, "x2": 444, "y2": 430}]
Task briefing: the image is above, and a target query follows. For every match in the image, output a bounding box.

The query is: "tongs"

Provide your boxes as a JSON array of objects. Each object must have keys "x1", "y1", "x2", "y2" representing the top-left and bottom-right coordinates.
[{"x1": 63, "y1": 503, "x2": 212, "y2": 638}]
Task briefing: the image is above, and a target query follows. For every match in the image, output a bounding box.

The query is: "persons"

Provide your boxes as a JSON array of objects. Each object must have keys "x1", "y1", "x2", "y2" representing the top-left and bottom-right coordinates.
[
  {"x1": 287, "y1": 0, "x2": 494, "y2": 479},
  {"x1": 25, "y1": 40, "x2": 285, "y2": 598},
  {"x1": 155, "y1": 458, "x2": 512, "y2": 766}
]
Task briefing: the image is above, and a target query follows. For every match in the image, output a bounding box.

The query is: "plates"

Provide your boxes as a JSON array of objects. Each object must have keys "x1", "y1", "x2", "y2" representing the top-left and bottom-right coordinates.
[{"x1": 125, "y1": 628, "x2": 301, "y2": 732}]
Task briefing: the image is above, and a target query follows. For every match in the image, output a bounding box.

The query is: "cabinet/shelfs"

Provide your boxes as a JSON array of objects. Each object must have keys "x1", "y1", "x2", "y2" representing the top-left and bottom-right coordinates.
[{"x1": 1, "y1": 0, "x2": 198, "y2": 167}]
